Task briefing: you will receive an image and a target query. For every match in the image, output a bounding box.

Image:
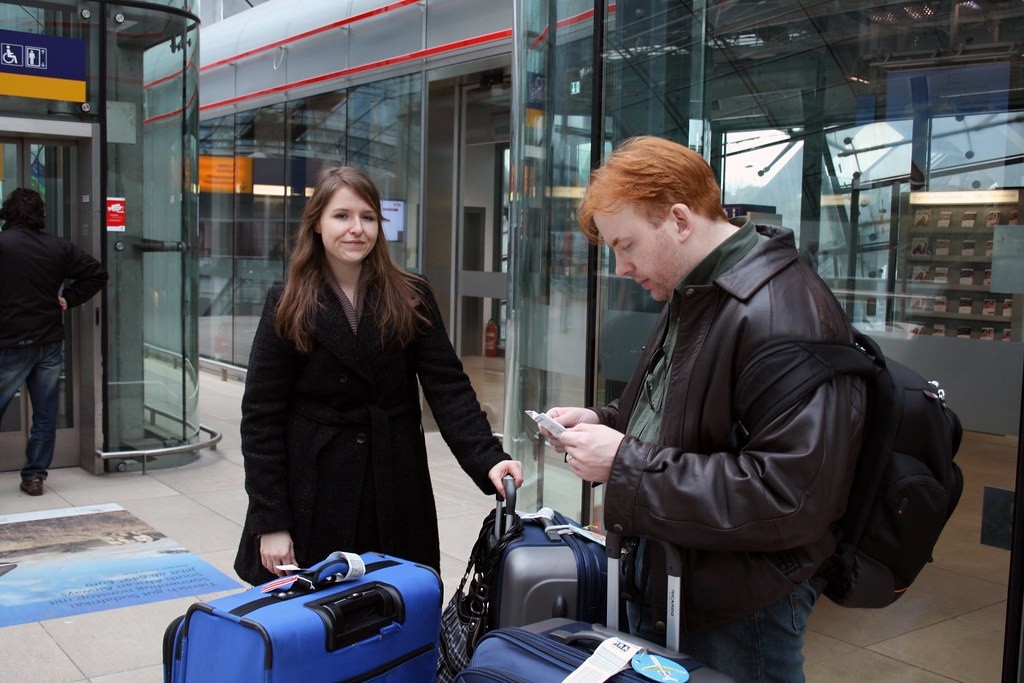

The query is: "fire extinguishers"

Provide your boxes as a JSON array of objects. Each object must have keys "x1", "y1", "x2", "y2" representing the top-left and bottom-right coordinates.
[{"x1": 486, "y1": 318, "x2": 498, "y2": 358}]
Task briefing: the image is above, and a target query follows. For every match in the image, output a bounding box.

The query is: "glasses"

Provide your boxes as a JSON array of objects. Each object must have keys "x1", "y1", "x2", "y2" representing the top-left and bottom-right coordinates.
[{"x1": 643, "y1": 344, "x2": 667, "y2": 414}]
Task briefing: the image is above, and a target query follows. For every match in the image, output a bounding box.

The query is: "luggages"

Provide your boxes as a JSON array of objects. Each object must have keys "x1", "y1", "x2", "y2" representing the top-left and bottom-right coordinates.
[
  {"x1": 454, "y1": 532, "x2": 737, "y2": 683},
  {"x1": 163, "y1": 552, "x2": 444, "y2": 683},
  {"x1": 488, "y1": 475, "x2": 608, "y2": 633}
]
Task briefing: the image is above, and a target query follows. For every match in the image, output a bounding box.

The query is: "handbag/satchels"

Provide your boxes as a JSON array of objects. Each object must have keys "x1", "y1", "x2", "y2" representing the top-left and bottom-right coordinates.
[{"x1": 437, "y1": 507, "x2": 525, "y2": 683}]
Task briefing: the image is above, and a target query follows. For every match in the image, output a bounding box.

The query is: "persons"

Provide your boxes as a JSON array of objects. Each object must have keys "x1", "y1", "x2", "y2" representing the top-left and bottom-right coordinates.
[
  {"x1": 233, "y1": 167, "x2": 524, "y2": 594},
  {"x1": 0, "y1": 188, "x2": 108, "y2": 497},
  {"x1": 538, "y1": 133, "x2": 866, "y2": 683}
]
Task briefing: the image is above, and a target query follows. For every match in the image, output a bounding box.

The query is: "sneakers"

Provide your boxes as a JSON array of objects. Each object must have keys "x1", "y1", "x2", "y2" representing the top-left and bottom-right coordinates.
[{"x1": 20, "y1": 478, "x2": 43, "y2": 496}]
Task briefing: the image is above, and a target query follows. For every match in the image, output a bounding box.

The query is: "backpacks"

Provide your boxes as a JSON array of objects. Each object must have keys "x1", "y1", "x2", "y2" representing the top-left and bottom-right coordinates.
[{"x1": 730, "y1": 331, "x2": 964, "y2": 609}]
